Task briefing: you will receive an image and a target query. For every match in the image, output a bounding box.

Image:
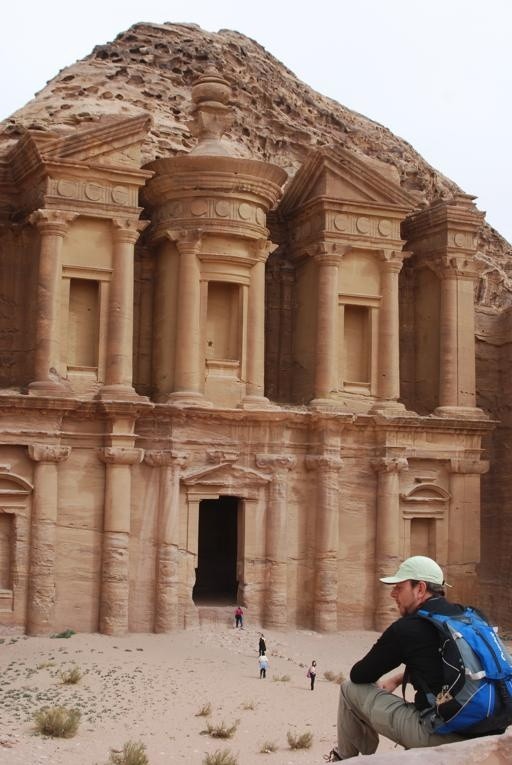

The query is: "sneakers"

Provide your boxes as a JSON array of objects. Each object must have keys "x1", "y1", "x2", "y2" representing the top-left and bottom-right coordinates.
[{"x1": 330, "y1": 749, "x2": 342, "y2": 761}]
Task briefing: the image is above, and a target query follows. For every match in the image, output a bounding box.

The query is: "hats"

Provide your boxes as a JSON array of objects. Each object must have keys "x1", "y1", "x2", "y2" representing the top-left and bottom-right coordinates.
[{"x1": 378, "y1": 555, "x2": 444, "y2": 585}]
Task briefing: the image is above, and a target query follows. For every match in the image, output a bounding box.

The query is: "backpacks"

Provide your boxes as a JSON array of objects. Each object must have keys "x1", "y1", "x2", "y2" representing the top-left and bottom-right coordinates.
[{"x1": 413, "y1": 604, "x2": 512, "y2": 737}]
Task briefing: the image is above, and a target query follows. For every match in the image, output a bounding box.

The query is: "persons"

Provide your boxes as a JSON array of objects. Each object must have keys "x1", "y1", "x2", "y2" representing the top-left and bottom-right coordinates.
[
  {"x1": 325, "y1": 556, "x2": 510, "y2": 759},
  {"x1": 233, "y1": 606, "x2": 317, "y2": 691}
]
